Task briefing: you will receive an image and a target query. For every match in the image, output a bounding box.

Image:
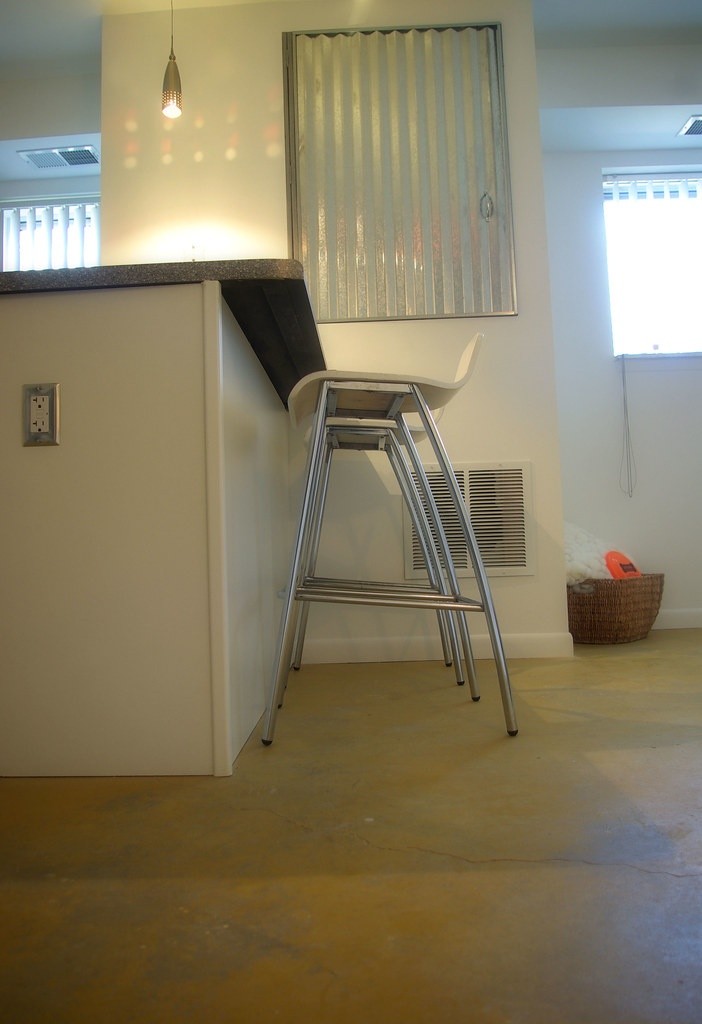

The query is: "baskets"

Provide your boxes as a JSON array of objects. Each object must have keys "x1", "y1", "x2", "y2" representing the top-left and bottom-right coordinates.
[{"x1": 566, "y1": 573, "x2": 664, "y2": 644}]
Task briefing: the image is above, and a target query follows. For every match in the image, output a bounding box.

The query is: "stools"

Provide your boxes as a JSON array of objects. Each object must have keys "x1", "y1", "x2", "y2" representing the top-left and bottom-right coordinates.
[
  {"x1": 261, "y1": 331, "x2": 520, "y2": 739},
  {"x1": 283, "y1": 405, "x2": 466, "y2": 689}
]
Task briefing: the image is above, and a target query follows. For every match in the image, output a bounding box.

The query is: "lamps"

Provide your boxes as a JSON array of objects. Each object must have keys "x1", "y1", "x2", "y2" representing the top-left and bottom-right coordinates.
[{"x1": 161, "y1": 0, "x2": 183, "y2": 119}]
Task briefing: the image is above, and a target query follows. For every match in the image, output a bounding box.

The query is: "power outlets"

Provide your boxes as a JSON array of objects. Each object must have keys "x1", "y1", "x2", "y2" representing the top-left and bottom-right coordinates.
[{"x1": 23, "y1": 383, "x2": 61, "y2": 447}]
